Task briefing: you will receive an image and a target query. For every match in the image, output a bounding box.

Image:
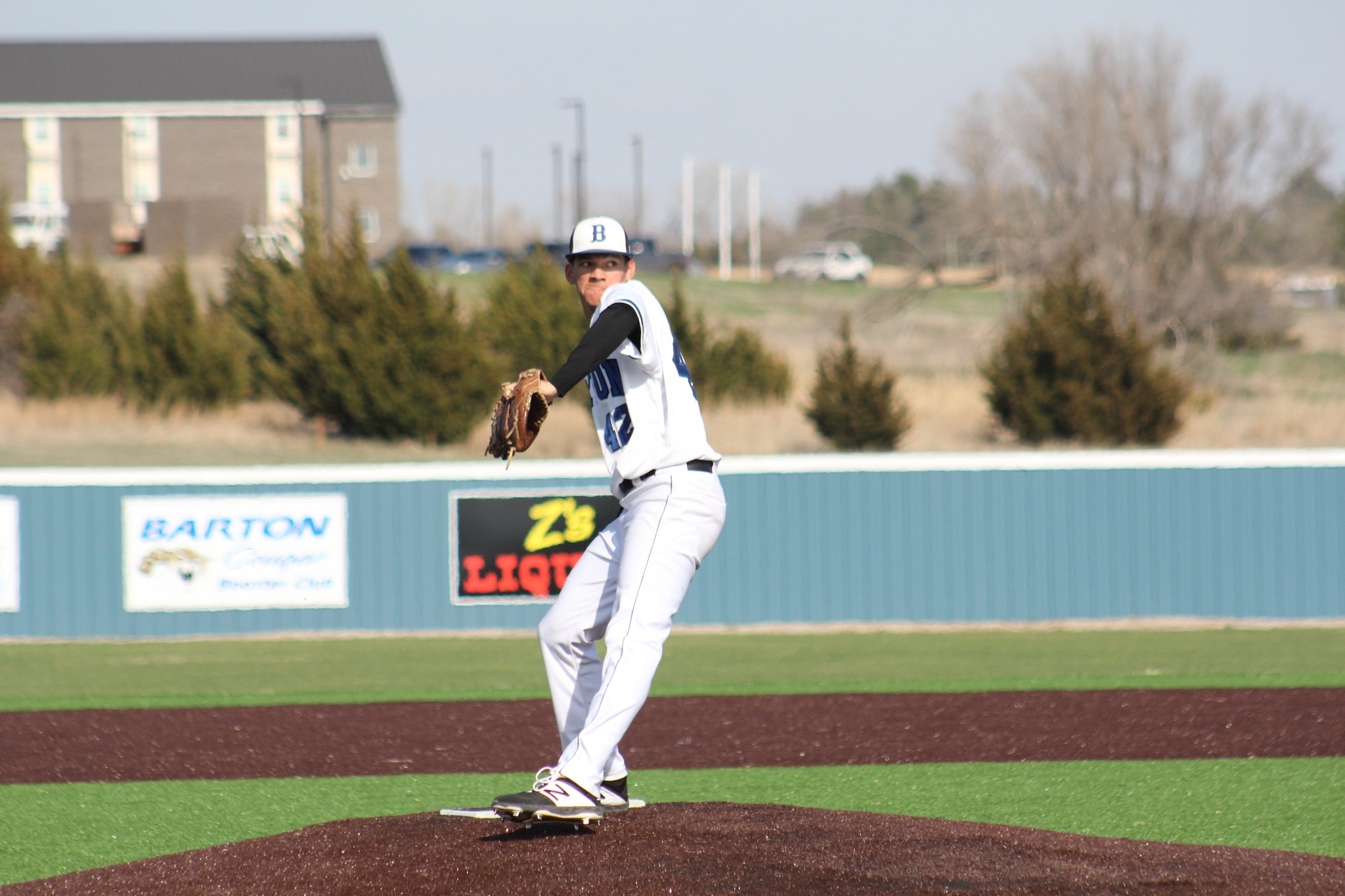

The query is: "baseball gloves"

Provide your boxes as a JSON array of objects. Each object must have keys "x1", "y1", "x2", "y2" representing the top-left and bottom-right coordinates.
[{"x1": 488, "y1": 367, "x2": 554, "y2": 461}]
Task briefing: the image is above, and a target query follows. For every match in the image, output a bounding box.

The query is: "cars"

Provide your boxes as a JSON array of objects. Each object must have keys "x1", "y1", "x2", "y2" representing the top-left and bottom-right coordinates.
[
  {"x1": 377, "y1": 237, "x2": 705, "y2": 278},
  {"x1": 773, "y1": 240, "x2": 872, "y2": 283}
]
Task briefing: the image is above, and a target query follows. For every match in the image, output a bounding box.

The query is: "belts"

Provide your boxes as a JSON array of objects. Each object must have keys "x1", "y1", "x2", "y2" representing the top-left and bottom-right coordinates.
[{"x1": 619, "y1": 459, "x2": 713, "y2": 497}]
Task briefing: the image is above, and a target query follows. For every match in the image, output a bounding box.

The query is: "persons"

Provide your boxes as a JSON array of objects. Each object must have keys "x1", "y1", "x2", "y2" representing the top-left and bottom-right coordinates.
[{"x1": 485, "y1": 217, "x2": 725, "y2": 826}]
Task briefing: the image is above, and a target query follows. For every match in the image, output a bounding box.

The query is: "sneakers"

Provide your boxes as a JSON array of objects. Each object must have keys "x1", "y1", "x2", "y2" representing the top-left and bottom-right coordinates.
[
  {"x1": 600, "y1": 776, "x2": 629, "y2": 813},
  {"x1": 491, "y1": 766, "x2": 603, "y2": 830}
]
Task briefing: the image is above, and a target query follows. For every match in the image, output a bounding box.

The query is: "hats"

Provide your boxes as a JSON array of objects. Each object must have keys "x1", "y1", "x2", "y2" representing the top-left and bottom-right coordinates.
[{"x1": 566, "y1": 217, "x2": 634, "y2": 265}]
